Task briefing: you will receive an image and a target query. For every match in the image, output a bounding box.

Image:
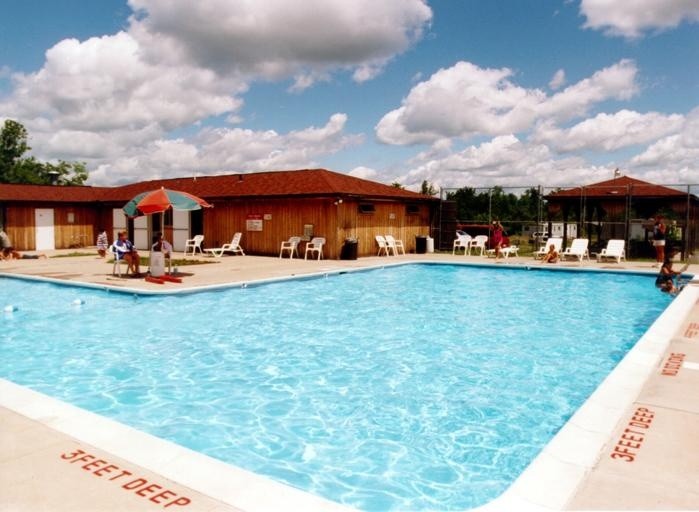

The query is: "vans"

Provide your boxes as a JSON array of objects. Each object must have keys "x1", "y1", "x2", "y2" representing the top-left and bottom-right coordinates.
[
  {"x1": 530, "y1": 231, "x2": 559, "y2": 245},
  {"x1": 456, "y1": 225, "x2": 510, "y2": 254}
]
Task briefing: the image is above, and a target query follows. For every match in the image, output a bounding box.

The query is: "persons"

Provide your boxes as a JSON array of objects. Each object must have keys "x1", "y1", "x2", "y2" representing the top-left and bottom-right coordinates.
[
  {"x1": 660, "y1": 278, "x2": 678, "y2": 294},
  {"x1": 0, "y1": 226, "x2": 49, "y2": 263},
  {"x1": 96, "y1": 228, "x2": 108, "y2": 258},
  {"x1": 541, "y1": 244, "x2": 558, "y2": 263},
  {"x1": 655, "y1": 258, "x2": 676, "y2": 288},
  {"x1": 650, "y1": 215, "x2": 666, "y2": 268},
  {"x1": 153, "y1": 233, "x2": 170, "y2": 258},
  {"x1": 112, "y1": 230, "x2": 140, "y2": 276},
  {"x1": 492, "y1": 224, "x2": 504, "y2": 260}
]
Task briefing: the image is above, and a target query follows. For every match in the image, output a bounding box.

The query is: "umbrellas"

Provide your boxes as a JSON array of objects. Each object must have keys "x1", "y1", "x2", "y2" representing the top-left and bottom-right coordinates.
[{"x1": 122, "y1": 185, "x2": 214, "y2": 239}]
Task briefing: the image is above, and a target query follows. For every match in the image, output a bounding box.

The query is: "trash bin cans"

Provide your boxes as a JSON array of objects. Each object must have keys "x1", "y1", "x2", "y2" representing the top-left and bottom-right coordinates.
[
  {"x1": 344, "y1": 240, "x2": 358, "y2": 260},
  {"x1": 417, "y1": 237, "x2": 434, "y2": 254}
]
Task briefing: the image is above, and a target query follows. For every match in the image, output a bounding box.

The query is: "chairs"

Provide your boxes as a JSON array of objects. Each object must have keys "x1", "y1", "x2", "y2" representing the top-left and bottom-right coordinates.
[
  {"x1": 204, "y1": 232, "x2": 246, "y2": 258},
  {"x1": 304, "y1": 237, "x2": 326, "y2": 261},
  {"x1": 109, "y1": 243, "x2": 136, "y2": 279},
  {"x1": 279, "y1": 236, "x2": 302, "y2": 259},
  {"x1": 533, "y1": 237, "x2": 565, "y2": 261},
  {"x1": 453, "y1": 234, "x2": 472, "y2": 256},
  {"x1": 468, "y1": 235, "x2": 489, "y2": 256},
  {"x1": 385, "y1": 235, "x2": 406, "y2": 257},
  {"x1": 375, "y1": 235, "x2": 396, "y2": 257},
  {"x1": 487, "y1": 244, "x2": 520, "y2": 260},
  {"x1": 595, "y1": 239, "x2": 628, "y2": 264},
  {"x1": 147, "y1": 239, "x2": 173, "y2": 275},
  {"x1": 184, "y1": 234, "x2": 205, "y2": 256},
  {"x1": 558, "y1": 237, "x2": 591, "y2": 263}
]
